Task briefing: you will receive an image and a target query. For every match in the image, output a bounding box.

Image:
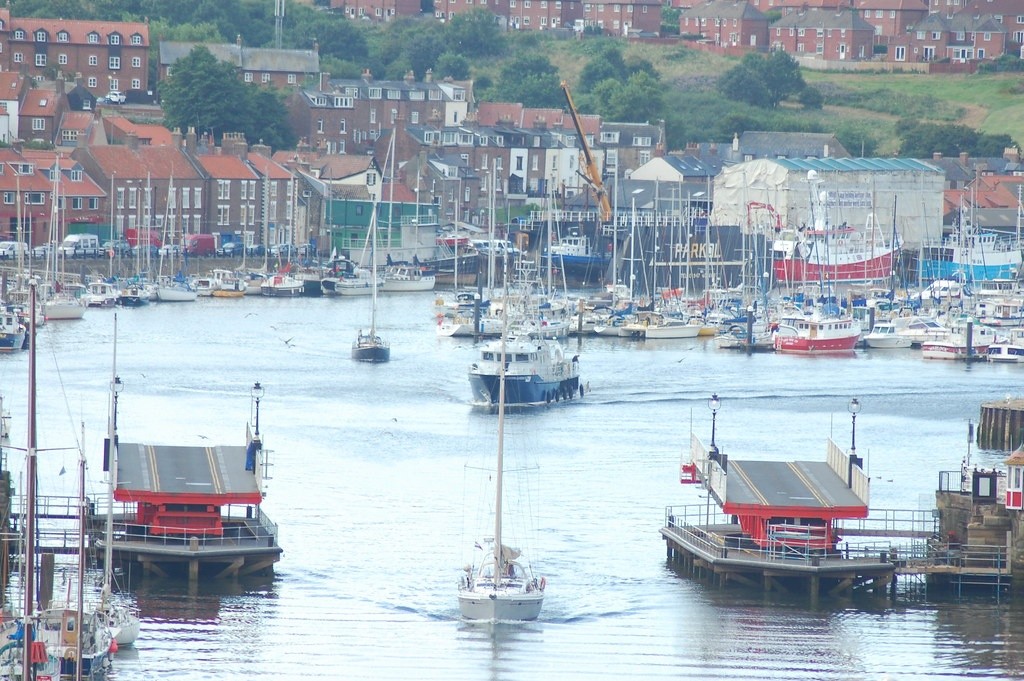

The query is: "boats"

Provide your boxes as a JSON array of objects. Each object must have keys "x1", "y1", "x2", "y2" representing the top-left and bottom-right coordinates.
[
  {"x1": 195, "y1": 256, "x2": 320, "y2": 298},
  {"x1": 465, "y1": 309, "x2": 586, "y2": 406}
]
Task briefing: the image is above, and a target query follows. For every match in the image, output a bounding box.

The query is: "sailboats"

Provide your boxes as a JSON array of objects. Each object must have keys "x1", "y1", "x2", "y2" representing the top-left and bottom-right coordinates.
[
  {"x1": 431, "y1": 158, "x2": 1024, "y2": 363},
  {"x1": 455, "y1": 285, "x2": 548, "y2": 620},
  {"x1": 319, "y1": 127, "x2": 436, "y2": 296},
  {"x1": 0, "y1": 150, "x2": 196, "y2": 350},
  {"x1": 350, "y1": 208, "x2": 394, "y2": 361},
  {"x1": 0, "y1": 391, "x2": 140, "y2": 681}
]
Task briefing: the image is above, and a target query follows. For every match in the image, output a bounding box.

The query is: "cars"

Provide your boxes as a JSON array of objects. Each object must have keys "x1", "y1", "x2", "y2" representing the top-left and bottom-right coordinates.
[{"x1": 0, "y1": 229, "x2": 316, "y2": 260}]
